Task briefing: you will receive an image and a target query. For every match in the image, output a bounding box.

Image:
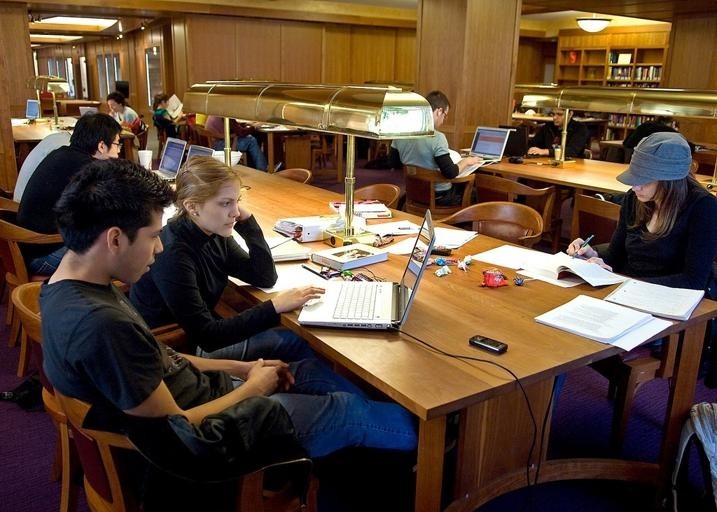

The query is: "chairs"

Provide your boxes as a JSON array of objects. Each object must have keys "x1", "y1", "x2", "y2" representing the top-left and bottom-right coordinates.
[
  {"x1": 438, "y1": 203, "x2": 543, "y2": 249},
  {"x1": 343, "y1": 183, "x2": 400, "y2": 207},
  {"x1": 195, "y1": 129, "x2": 248, "y2": 165},
  {"x1": 312, "y1": 133, "x2": 337, "y2": 178},
  {"x1": 37, "y1": 327, "x2": 319, "y2": 512},
  {"x1": 574, "y1": 195, "x2": 629, "y2": 243},
  {"x1": 402, "y1": 162, "x2": 477, "y2": 219},
  {"x1": 0, "y1": 201, "x2": 69, "y2": 284},
  {"x1": 134, "y1": 125, "x2": 150, "y2": 151},
  {"x1": 10, "y1": 279, "x2": 188, "y2": 390},
  {"x1": 272, "y1": 168, "x2": 311, "y2": 185},
  {"x1": 476, "y1": 173, "x2": 562, "y2": 253},
  {"x1": 156, "y1": 123, "x2": 173, "y2": 156},
  {"x1": 180, "y1": 125, "x2": 209, "y2": 157}
]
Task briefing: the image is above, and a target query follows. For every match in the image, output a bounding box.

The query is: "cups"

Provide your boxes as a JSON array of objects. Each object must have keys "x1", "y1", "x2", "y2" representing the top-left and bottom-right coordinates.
[{"x1": 138, "y1": 149, "x2": 154, "y2": 169}]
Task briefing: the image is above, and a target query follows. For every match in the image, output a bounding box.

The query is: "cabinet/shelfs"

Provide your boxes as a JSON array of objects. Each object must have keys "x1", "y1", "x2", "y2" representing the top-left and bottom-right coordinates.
[{"x1": 553, "y1": 31, "x2": 669, "y2": 148}]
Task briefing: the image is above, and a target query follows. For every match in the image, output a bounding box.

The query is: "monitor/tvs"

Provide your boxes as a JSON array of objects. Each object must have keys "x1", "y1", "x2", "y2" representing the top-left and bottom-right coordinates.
[{"x1": 115, "y1": 80, "x2": 129, "y2": 98}]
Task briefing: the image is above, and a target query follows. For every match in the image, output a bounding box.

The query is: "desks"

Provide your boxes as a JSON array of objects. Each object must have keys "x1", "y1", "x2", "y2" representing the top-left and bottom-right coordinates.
[
  {"x1": 512, "y1": 115, "x2": 607, "y2": 149},
  {"x1": 453, "y1": 156, "x2": 717, "y2": 243},
  {"x1": 236, "y1": 118, "x2": 342, "y2": 183},
  {"x1": 142, "y1": 159, "x2": 717, "y2": 511},
  {"x1": 13, "y1": 116, "x2": 136, "y2": 177}
]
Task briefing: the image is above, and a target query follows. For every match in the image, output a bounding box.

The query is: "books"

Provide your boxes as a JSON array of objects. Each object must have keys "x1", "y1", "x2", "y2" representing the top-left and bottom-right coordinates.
[
  {"x1": 525, "y1": 251, "x2": 629, "y2": 285},
  {"x1": 165, "y1": 93, "x2": 184, "y2": 118},
  {"x1": 634, "y1": 81, "x2": 658, "y2": 87},
  {"x1": 440, "y1": 148, "x2": 485, "y2": 178},
  {"x1": 610, "y1": 66, "x2": 632, "y2": 80},
  {"x1": 312, "y1": 243, "x2": 388, "y2": 271},
  {"x1": 329, "y1": 199, "x2": 392, "y2": 221},
  {"x1": 636, "y1": 67, "x2": 661, "y2": 81},
  {"x1": 608, "y1": 114, "x2": 655, "y2": 127},
  {"x1": 607, "y1": 81, "x2": 630, "y2": 86}
]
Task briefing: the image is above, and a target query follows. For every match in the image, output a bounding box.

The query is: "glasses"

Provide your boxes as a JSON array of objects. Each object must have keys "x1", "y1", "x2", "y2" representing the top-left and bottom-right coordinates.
[
  {"x1": 442, "y1": 111, "x2": 449, "y2": 120},
  {"x1": 550, "y1": 112, "x2": 564, "y2": 116},
  {"x1": 113, "y1": 142, "x2": 123, "y2": 149}
]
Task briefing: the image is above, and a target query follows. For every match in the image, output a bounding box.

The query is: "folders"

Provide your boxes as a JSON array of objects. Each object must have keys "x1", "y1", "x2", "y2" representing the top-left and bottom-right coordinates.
[{"x1": 273, "y1": 213, "x2": 366, "y2": 242}]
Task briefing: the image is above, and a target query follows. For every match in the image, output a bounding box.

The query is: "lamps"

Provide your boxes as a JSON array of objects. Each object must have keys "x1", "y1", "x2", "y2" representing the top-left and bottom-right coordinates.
[
  {"x1": 182, "y1": 79, "x2": 437, "y2": 248},
  {"x1": 36, "y1": 75, "x2": 69, "y2": 130},
  {"x1": 513, "y1": 85, "x2": 717, "y2": 169},
  {"x1": 574, "y1": 14, "x2": 612, "y2": 34}
]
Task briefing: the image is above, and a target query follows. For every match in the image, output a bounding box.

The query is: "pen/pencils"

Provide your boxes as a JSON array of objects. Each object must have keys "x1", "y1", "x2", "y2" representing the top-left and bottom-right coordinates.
[
  {"x1": 572, "y1": 235, "x2": 594, "y2": 258},
  {"x1": 397, "y1": 227, "x2": 420, "y2": 230},
  {"x1": 270, "y1": 238, "x2": 293, "y2": 250},
  {"x1": 303, "y1": 265, "x2": 327, "y2": 279}
]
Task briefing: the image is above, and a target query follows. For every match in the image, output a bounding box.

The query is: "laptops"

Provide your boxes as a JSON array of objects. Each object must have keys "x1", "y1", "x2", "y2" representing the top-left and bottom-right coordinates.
[
  {"x1": 461, "y1": 126, "x2": 510, "y2": 163},
  {"x1": 79, "y1": 107, "x2": 98, "y2": 117},
  {"x1": 152, "y1": 137, "x2": 188, "y2": 182},
  {"x1": 260, "y1": 124, "x2": 280, "y2": 129},
  {"x1": 499, "y1": 125, "x2": 540, "y2": 159},
  {"x1": 297, "y1": 209, "x2": 436, "y2": 330},
  {"x1": 186, "y1": 144, "x2": 215, "y2": 163},
  {"x1": 26, "y1": 100, "x2": 41, "y2": 119}
]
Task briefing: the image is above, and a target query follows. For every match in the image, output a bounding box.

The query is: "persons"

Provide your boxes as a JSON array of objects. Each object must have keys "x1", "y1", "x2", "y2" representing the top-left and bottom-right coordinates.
[
  {"x1": 203, "y1": 115, "x2": 282, "y2": 175},
  {"x1": 13, "y1": 130, "x2": 72, "y2": 202},
  {"x1": 152, "y1": 93, "x2": 185, "y2": 140},
  {"x1": 388, "y1": 90, "x2": 484, "y2": 229},
  {"x1": 528, "y1": 106, "x2": 587, "y2": 159},
  {"x1": 17, "y1": 111, "x2": 122, "y2": 269},
  {"x1": 566, "y1": 132, "x2": 717, "y2": 300},
  {"x1": 38, "y1": 157, "x2": 459, "y2": 508},
  {"x1": 105, "y1": 91, "x2": 141, "y2": 135},
  {"x1": 623, "y1": 116, "x2": 696, "y2": 163},
  {"x1": 131, "y1": 154, "x2": 326, "y2": 363}
]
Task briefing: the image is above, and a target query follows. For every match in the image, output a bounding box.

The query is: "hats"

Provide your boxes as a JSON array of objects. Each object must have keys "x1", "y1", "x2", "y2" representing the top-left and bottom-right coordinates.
[{"x1": 616, "y1": 131, "x2": 692, "y2": 187}]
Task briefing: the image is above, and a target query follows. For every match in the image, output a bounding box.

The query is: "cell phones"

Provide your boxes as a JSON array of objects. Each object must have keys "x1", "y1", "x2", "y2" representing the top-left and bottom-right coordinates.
[
  {"x1": 431, "y1": 249, "x2": 452, "y2": 256},
  {"x1": 469, "y1": 335, "x2": 508, "y2": 354}
]
